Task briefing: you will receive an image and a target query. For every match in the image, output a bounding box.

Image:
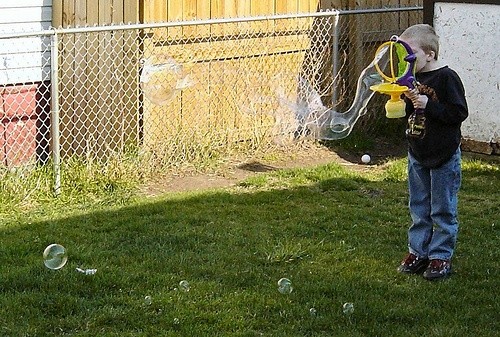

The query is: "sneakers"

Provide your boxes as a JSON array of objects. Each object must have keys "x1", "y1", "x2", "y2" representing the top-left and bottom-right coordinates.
[
  {"x1": 396, "y1": 252, "x2": 429, "y2": 275},
  {"x1": 422, "y1": 259, "x2": 453, "y2": 279}
]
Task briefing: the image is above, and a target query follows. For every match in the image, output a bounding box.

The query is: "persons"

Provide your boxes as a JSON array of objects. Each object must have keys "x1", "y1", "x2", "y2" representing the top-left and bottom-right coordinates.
[{"x1": 394, "y1": 22, "x2": 470, "y2": 283}]
